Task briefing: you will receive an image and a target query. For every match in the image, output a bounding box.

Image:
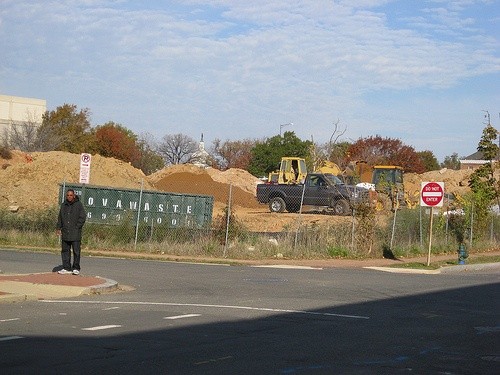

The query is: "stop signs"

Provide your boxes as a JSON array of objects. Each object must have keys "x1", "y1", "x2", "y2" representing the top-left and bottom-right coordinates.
[{"x1": 420, "y1": 181, "x2": 444, "y2": 206}]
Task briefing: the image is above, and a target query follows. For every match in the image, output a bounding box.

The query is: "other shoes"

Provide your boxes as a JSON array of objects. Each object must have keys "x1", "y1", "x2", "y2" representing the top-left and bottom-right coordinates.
[
  {"x1": 58, "y1": 268, "x2": 73, "y2": 275},
  {"x1": 73, "y1": 269, "x2": 79, "y2": 275}
]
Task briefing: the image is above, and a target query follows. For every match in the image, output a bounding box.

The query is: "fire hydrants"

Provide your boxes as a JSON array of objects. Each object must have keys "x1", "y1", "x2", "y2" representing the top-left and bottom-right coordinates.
[{"x1": 457, "y1": 243, "x2": 469, "y2": 265}]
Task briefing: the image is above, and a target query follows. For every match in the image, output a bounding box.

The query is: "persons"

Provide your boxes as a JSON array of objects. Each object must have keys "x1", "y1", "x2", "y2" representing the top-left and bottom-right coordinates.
[{"x1": 56, "y1": 189, "x2": 87, "y2": 275}]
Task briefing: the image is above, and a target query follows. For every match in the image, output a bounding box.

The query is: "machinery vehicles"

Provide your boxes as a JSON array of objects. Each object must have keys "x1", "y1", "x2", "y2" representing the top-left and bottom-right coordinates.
[{"x1": 268, "y1": 157, "x2": 470, "y2": 214}]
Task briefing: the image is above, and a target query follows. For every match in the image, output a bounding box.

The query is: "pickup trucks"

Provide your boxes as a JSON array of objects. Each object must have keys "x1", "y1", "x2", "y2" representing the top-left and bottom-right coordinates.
[{"x1": 257, "y1": 173, "x2": 369, "y2": 216}]
